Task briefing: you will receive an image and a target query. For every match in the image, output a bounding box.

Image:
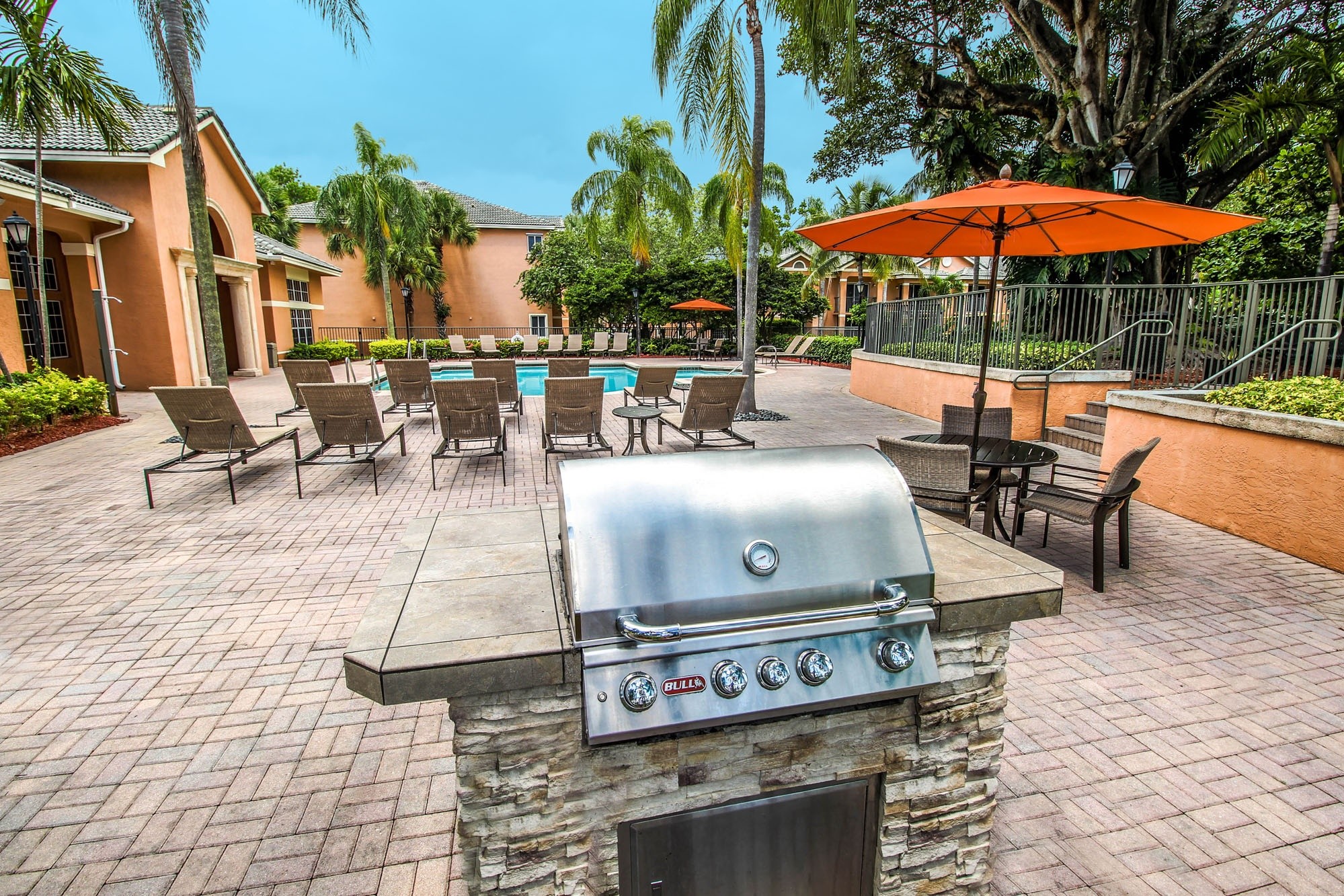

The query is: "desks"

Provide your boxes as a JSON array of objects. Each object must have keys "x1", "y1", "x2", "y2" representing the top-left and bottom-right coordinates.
[
  {"x1": 432, "y1": 347, "x2": 447, "y2": 361},
  {"x1": 687, "y1": 342, "x2": 710, "y2": 361},
  {"x1": 899, "y1": 433, "x2": 1059, "y2": 541},
  {"x1": 506, "y1": 346, "x2": 520, "y2": 359},
  {"x1": 615, "y1": 406, "x2": 662, "y2": 455}
]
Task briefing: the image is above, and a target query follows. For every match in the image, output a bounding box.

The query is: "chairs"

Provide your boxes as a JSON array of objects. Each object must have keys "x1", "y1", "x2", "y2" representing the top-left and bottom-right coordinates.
[
  {"x1": 143, "y1": 386, "x2": 301, "y2": 509},
  {"x1": 296, "y1": 382, "x2": 407, "y2": 499},
  {"x1": 429, "y1": 377, "x2": 507, "y2": 490},
  {"x1": 875, "y1": 404, "x2": 1160, "y2": 593},
  {"x1": 448, "y1": 335, "x2": 475, "y2": 362},
  {"x1": 471, "y1": 359, "x2": 524, "y2": 433},
  {"x1": 542, "y1": 359, "x2": 611, "y2": 483},
  {"x1": 479, "y1": 335, "x2": 502, "y2": 360},
  {"x1": 624, "y1": 365, "x2": 756, "y2": 453},
  {"x1": 520, "y1": 332, "x2": 628, "y2": 360},
  {"x1": 693, "y1": 339, "x2": 724, "y2": 362},
  {"x1": 382, "y1": 358, "x2": 436, "y2": 435},
  {"x1": 275, "y1": 359, "x2": 335, "y2": 427},
  {"x1": 754, "y1": 336, "x2": 822, "y2": 367}
]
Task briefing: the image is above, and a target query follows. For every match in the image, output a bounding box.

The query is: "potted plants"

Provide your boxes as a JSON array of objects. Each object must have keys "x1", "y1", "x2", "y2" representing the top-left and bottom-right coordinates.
[{"x1": 1201, "y1": 294, "x2": 1295, "y2": 385}]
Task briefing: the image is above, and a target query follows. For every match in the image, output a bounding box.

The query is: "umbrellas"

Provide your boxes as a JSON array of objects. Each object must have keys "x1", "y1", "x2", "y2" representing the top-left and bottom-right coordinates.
[
  {"x1": 794, "y1": 163, "x2": 1267, "y2": 485},
  {"x1": 670, "y1": 297, "x2": 734, "y2": 359}
]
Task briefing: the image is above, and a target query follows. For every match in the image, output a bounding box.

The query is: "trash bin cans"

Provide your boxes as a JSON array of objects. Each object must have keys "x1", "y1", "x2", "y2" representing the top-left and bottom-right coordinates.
[
  {"x1": 267, "y1": 343, "x2": 278, "y2": 368},
  {"x1": 1121, "y1": 311, "x2": 1174, "y2": 375}
]
